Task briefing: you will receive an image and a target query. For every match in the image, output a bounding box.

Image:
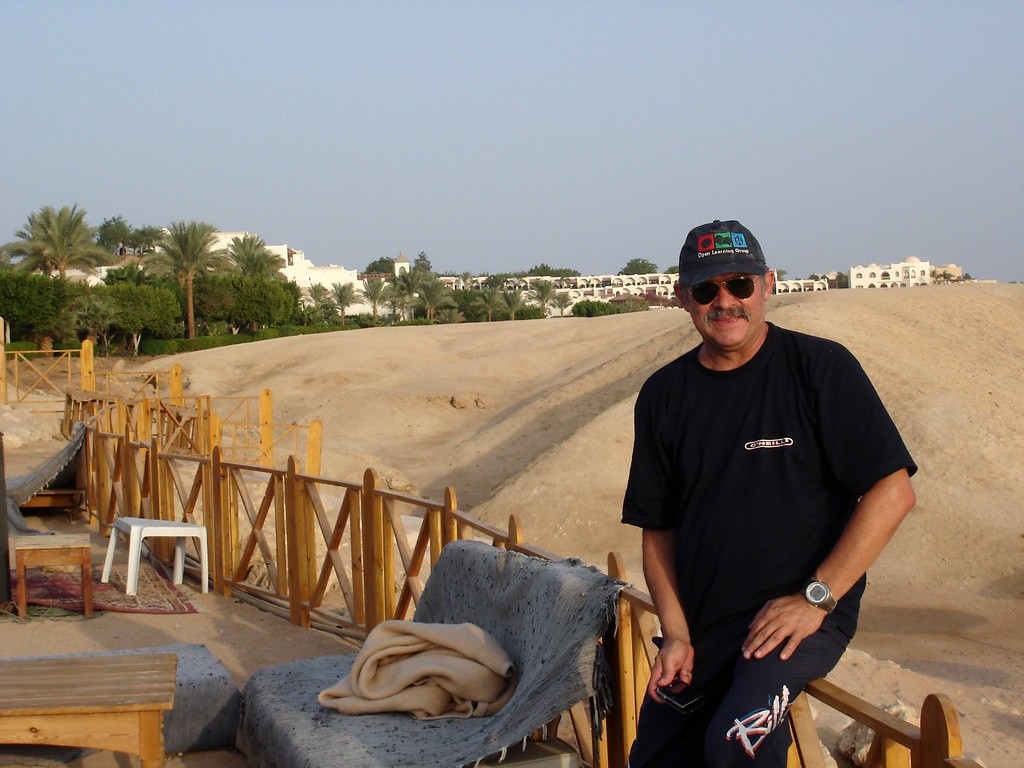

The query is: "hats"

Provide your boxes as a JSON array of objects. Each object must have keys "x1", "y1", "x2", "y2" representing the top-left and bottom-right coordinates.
[{"x1": 678, "y1": 220, "x2": 766, "y2": 287}]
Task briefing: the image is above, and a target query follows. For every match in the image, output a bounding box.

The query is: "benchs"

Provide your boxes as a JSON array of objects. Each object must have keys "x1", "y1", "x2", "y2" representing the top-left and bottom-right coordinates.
[
  {"x1": 0, "y1": 644, "x2": 241, "y2": 756},
  {"x1": 240, "y1": 539, "x2": 634, "y2": 768},
  {"x1": 18, "y1": 489, "x2": 86, "y2": 525}
]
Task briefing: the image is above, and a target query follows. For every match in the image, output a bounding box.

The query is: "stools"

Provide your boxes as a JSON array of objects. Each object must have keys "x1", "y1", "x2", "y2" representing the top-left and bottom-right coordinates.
[
  {"x1": 101, "y1": 518, "x2": 208, "y2": 596},
  {"x1": 15, "y1": 533, "x2": 92, "y2": 618}
]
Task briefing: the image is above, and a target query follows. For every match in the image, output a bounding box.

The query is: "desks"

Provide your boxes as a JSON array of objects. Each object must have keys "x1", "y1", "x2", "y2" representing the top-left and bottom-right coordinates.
[{"x1": 0, "y1": 654, "x2": 178, "y2": 768}]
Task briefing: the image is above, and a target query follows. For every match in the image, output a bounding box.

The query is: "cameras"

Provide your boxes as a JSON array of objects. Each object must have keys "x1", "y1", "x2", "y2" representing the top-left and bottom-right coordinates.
[{"x1": 656, "y1": 677, "x2": 704, "y2": 714}]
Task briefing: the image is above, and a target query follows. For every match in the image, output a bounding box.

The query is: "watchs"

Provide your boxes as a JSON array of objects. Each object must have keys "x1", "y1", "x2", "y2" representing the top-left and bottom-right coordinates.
[{"x1": 801, "y1": 576, "x2": 838, "y2": 614}]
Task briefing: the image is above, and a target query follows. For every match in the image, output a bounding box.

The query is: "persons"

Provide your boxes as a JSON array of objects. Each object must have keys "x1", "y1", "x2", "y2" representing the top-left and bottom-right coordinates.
[{"x1": 621, "y1": 220, "x2": 918, "y2": 768}]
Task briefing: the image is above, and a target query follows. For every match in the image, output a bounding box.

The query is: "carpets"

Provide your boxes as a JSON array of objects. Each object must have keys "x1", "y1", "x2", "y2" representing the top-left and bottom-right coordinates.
[{"x1": 10, "y1": 563, "x2": 198, "y2": 614}]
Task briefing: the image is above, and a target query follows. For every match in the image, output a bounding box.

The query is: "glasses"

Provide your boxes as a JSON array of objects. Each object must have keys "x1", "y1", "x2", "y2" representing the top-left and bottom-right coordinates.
[{"x1": 685, "y1": 271, "x2": 763, "y2": 305}]
